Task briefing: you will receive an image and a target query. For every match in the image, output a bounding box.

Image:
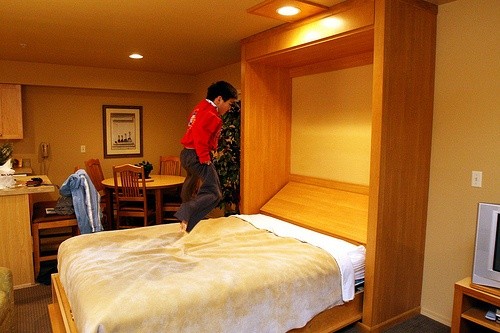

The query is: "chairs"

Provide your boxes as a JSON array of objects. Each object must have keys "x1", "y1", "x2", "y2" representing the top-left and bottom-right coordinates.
[
  {"x1": 85, "y1": 156, "x2": 202, "y2": 231},
  {"x1": 32, "y1": 169, "x2": 104, "y2": 279}
]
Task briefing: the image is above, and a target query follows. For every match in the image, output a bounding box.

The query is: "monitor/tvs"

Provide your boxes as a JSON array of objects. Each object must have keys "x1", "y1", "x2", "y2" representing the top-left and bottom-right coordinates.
[{"x1": 472, "y1": 202, "x2": 500, "y2": 288}]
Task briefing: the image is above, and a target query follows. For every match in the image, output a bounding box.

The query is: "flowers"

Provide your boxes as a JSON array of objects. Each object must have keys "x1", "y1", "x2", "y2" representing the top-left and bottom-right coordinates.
[{"x1": 212, "y1": 101, "x2": 241, "y2": 217}]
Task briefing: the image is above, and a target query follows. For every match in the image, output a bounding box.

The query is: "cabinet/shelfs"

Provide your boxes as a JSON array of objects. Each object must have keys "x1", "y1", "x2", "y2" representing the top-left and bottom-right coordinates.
[
  {"x1": 450, "y1": 276, "x2": 500, "y2": 333},
  {"x1": 0, "y1": 84, "x2": 24, "y2": 139}
]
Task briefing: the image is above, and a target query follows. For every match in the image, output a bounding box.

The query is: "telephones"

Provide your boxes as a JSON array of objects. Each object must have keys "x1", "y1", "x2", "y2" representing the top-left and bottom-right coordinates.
[{"x1": 40, "y1": 142, "x2": 49, "y2": 158}]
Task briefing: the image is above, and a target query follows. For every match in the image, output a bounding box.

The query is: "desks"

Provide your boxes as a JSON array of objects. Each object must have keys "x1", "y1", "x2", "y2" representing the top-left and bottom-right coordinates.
[
  {"x1": 0, "y1": 174, "x2": 54, "y2": 290},
  {"x1": 11, "y1": 167, "x2": 33, "y2": 174},
  {"x1": 102, "y1": 175, "x2": 186, "y2": 225}
]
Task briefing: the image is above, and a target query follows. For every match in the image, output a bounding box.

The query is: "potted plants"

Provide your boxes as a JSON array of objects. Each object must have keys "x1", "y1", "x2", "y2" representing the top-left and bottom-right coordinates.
[{"x1": 135, "y1": 161, "x2": 153, "y2": 178}]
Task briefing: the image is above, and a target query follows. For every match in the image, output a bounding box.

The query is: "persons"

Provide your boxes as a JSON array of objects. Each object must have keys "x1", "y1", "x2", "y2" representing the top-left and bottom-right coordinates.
[{"x1": 174, "y1": 80, "x2": 238, "y2": 234}]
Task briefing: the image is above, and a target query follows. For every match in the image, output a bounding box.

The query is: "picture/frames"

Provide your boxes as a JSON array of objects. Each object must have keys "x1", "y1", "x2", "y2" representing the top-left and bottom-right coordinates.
[{"x1": 102, "y1": 104, "x2": 143, "y2": 159}]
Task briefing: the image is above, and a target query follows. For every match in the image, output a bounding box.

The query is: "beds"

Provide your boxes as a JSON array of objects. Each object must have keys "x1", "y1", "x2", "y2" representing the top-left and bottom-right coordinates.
[{"x1": 48, "y1": 181, "x2": 370, "y2": 333}]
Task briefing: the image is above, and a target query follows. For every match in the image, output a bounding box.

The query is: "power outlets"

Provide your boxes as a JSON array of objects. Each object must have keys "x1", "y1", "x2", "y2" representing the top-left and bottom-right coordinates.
[{"x1": 471, "y1": 171, "x2": 482, "y2": 188}]
